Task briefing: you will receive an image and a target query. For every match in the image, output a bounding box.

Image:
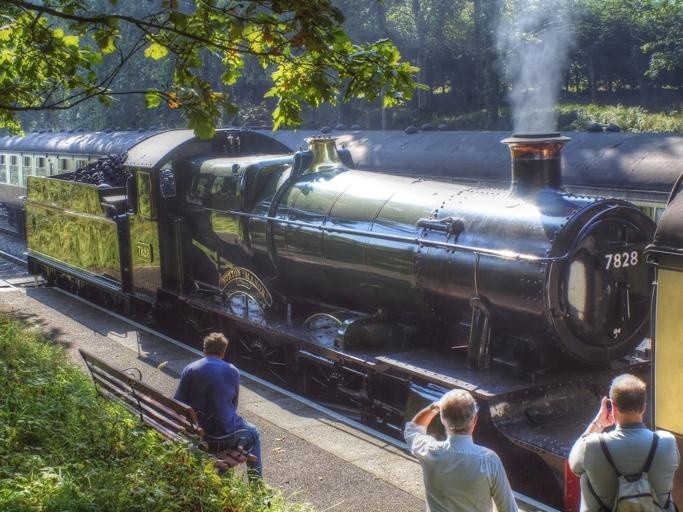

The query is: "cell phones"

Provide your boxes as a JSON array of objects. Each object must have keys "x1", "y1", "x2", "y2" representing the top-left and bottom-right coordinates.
[{"x1": 607, "y1": 400, "x2": 612, "y2": 414}]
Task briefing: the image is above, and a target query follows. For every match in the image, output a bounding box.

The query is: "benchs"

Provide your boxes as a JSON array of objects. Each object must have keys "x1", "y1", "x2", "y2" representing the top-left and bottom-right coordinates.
[{"x1": 75, "y1": 348, "x2": 257, "y2": 477}]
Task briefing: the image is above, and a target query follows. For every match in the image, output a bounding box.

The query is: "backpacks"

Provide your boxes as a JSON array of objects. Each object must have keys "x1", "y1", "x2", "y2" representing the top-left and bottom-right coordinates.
[{"x1": 586, "y1": 432, "x2": 673, "y2": 511}]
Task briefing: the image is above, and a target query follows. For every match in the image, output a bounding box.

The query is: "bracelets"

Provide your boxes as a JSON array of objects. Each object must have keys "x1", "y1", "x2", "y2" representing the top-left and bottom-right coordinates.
[
  {"x1": 430, "y1": 402, "x2": 440, "y2": 415},
  {"x1": 592, "y1": 419, "x2": 605, "y2": 431}
]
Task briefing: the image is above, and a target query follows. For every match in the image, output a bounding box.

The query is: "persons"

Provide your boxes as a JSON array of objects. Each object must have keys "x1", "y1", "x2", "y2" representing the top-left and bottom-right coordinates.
[
  {"x1": 404, "y1": 388, "x2": 519, "y2": 511},
  {"x1": 568, "y1": 374, "x2": 681, "y2": 512},
  {"x1": 173, "y1": 332, "x2": 261, "y2": 493}
]
